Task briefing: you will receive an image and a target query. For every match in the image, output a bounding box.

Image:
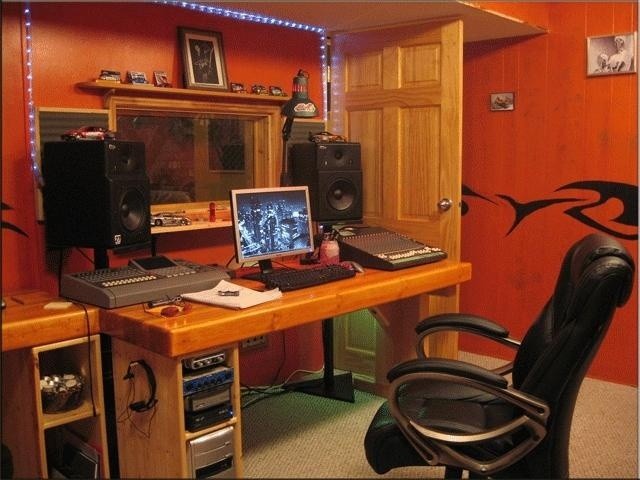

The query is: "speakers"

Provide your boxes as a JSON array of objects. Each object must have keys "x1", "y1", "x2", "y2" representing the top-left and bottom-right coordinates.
[
  {"x1": 292, "y1": 142, "x2": 364, "y2": 226},
  {"x1": 42, "y1": 141, "x2": 153, "y2": 254}
]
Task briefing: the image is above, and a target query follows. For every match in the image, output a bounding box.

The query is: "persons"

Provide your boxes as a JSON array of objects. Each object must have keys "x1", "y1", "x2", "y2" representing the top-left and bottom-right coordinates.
[
  {"x1": 608, "y1": 35, "x2": 631, "y2": 73},
  {"x1": 593, "y1": 54, "x2": 610, "y2": 74}
]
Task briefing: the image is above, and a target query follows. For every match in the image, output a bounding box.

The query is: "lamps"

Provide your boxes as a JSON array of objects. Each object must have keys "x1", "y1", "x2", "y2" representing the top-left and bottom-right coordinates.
[{"x1": 282, "y1": 66, "x2": 319, "y2": 144}]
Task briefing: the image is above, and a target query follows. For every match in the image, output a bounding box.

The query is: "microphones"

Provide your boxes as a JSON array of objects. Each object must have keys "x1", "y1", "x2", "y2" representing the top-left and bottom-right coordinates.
[{"x1": 139, "y1": 400, "x2": 159, "y2": 418}]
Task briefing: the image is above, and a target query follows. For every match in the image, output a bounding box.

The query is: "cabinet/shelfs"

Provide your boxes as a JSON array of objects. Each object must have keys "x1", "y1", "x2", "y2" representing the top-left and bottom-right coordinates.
[
  {"x1": 111, "y1": 338, "x2": 243, "y2": 480},
  {"x1": 1, "y1": 335, "x2": 110, "y2": 479}
]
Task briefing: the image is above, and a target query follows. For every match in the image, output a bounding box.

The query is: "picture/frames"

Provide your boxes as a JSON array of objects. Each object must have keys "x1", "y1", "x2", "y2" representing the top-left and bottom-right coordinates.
[
  {"x1": 585, "y1": 31, "x2": 638, "y2": 77},
  {"x1": 176, "y1": 25, "x2": 230, "y2": 92}
]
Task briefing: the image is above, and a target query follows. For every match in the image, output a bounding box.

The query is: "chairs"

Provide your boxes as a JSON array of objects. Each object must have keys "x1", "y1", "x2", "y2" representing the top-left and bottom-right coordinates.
[{"x1": 365, "y1": 233, "x2": 636, "y2": 479}]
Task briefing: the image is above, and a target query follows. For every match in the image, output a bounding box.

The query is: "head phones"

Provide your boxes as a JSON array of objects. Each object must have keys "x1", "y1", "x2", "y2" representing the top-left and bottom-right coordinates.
[{"x1": 121, "y1": 360, "x2": 157, "y2": 411}]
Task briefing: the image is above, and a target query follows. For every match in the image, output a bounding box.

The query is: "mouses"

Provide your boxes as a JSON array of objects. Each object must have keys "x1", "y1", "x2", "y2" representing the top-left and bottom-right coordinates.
[{"x1": 339, "y1": 260, "x2": 365, "y2": 273}]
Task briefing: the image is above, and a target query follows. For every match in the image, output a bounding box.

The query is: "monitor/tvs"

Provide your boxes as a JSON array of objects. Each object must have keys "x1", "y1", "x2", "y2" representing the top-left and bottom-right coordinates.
[{"x1": 229, "y1": 186, "x2": 314, "y2": 281}]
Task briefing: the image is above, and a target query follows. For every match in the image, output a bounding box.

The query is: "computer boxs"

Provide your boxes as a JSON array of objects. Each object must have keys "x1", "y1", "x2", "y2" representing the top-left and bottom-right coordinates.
[{"x1": 187, "y1": 424, "x2": 236, "y2": 480}]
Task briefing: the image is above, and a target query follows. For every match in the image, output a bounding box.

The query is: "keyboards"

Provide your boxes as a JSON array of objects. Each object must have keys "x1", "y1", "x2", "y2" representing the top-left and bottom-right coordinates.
[{"x1": 266, "y1": 264, "x2": 356, "y2": 292}]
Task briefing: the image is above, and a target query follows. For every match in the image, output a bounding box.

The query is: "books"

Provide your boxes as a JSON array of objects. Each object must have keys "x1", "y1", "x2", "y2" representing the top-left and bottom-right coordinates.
[
  {"x1": 47, "y1": 429, "x2": 103, "y2": 480},
  {"x1": 179, "y1": 278, "x2": 284, "y2": 311}
]
